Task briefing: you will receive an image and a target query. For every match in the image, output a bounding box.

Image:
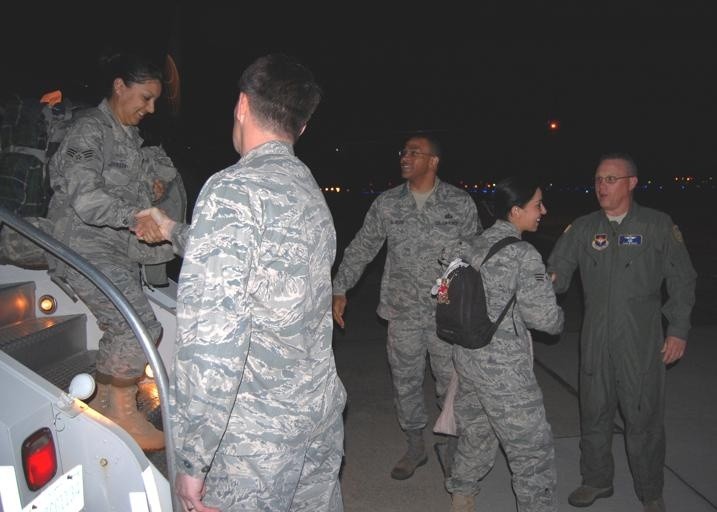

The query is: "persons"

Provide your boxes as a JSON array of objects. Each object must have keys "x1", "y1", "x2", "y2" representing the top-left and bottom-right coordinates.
[
  {"x1": 40, "y1": 38, "x2": 187, "y2": 454},
  {"x1": 441, "y1": 171, "x2": 566, "y2": 512},
  {"x1": 541, "y1": 149, "x2": 701, "y2": 511},
  {"x1": 128, "y1": 52, "x2": 348, "y2": 512},
  {"x1": 330, "y1": 133, "x2": 484, "y2": 482}
]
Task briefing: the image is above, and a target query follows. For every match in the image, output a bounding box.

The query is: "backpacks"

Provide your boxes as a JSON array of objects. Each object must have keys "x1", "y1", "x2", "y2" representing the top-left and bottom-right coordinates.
[{"x1": 430, "y1": 237, "x2": 521, "y2": 350}]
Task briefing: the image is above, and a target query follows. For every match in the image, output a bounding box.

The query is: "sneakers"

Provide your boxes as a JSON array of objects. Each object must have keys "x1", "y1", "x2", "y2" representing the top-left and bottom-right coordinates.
[
  {"x1": 643, "y1": 496, "x2": 666, "y2": 512},
  {"x1": 568, "y1": 484, "x2": 614, "y2": 507}
]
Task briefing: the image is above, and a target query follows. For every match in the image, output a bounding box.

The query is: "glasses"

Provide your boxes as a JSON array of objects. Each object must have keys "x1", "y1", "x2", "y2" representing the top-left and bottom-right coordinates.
[
  {"x1": 398, "y1": 149, "x2": 431, "y2": 158},
  {"x1": 592, "y1": 175, "x2": 635, "y2": 185}
]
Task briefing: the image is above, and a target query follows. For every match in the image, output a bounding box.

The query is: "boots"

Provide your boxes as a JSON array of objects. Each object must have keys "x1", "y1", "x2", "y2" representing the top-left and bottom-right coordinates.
[
  {"x1": 448, "y1": 493, "x2": 477, "y2": 512},
  {"x1": 87, "y1": 381, "x2": 111, "y2": 416},
  {"x1": 107, "y1": 383, "x2": 165, "y2": 452},
  {"x1": 391, "y1": 429, "x2": 428, "y2": 480}
]
